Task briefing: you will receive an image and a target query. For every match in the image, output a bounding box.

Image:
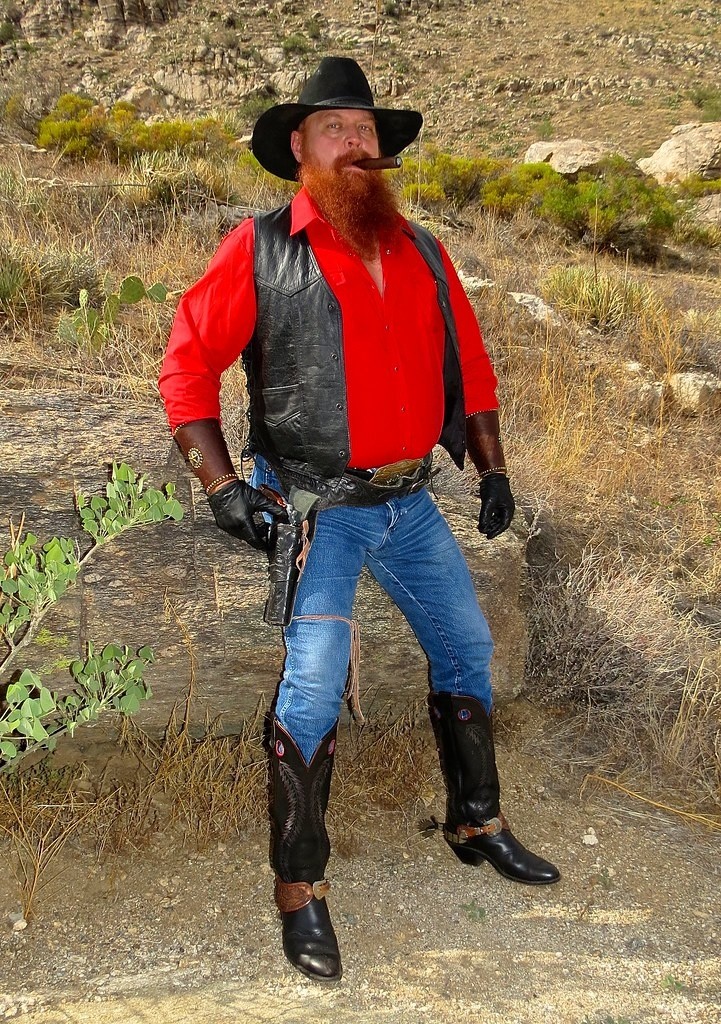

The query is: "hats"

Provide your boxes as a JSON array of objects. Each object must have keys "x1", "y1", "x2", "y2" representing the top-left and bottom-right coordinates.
[{"x1": 252, "y1": 56, "x2": 424, "y2": 182}]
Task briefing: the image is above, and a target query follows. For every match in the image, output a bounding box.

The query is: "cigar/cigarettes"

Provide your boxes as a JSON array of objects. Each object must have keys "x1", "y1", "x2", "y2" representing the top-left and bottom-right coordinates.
[{"x1": 353, "y1": 157, "x2": 403, "y2": 170}]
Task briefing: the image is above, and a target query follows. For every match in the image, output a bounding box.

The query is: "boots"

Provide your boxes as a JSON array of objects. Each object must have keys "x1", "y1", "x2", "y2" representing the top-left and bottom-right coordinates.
[
  {"x1": 262, "y1": 712, "x2": 344, "y2": 982},
  {"x1": 427, "y1": 691, "x2": 562, "y2": 885}
]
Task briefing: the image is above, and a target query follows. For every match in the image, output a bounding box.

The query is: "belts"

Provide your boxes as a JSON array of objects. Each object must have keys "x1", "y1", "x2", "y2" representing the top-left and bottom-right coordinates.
[{"x1": 359, "y1": 479, "x2": 427, "y2": 506}]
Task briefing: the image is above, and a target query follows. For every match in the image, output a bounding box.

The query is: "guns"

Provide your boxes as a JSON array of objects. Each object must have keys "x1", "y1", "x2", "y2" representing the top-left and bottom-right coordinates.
[{"x1": 251, "y1": 483, "x2": 312, "y2": 625}]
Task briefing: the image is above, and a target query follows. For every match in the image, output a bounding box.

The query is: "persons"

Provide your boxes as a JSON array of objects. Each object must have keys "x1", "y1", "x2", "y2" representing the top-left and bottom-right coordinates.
[{"x1": 157, "y1": 56, "x2": 559, "y2": 981}]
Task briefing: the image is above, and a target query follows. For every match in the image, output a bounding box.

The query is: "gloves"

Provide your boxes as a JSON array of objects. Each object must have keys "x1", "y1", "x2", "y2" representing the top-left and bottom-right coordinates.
[
  {"x1": 206, "y1": 479, "x2": 287, "y2": 549},
  {"x1": 476, "y1": 473, "x2": 515, "y2": 540}
]
